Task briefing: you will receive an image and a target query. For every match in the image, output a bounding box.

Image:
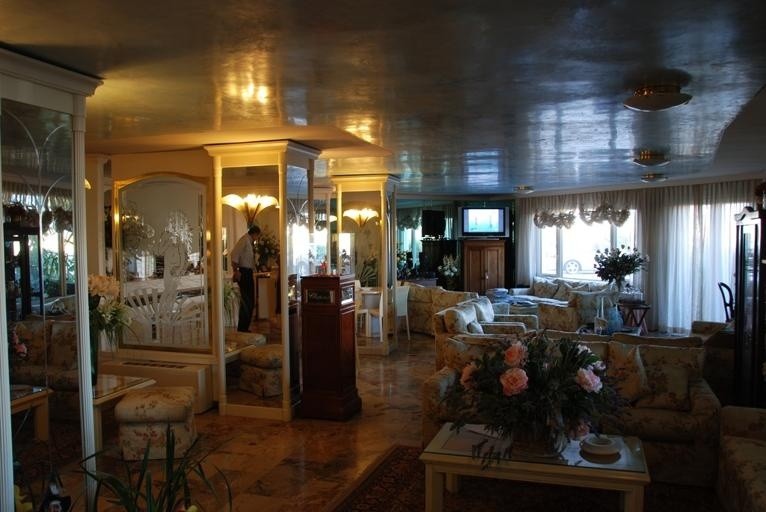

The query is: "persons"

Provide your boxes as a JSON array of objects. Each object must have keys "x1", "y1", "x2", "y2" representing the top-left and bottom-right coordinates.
[{"x1": 231, "y1": 225, "x2": 261, "y2": 334}]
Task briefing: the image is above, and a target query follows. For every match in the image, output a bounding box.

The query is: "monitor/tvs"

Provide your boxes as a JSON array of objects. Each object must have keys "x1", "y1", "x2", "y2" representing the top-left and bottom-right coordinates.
[{"x1": 458, "y1": 204, "x2": 511, "y2": 241}]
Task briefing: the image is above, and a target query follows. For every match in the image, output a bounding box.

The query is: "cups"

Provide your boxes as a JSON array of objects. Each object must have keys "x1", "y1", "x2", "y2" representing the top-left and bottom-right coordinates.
[{"x1": 588, "y1": 282, "x2": 593, "y2": 292}]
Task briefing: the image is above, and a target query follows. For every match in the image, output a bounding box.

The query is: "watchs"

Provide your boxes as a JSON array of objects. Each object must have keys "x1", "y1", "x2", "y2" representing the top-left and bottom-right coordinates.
[{"x1": 233, "y1": 267, "x2": 240, "y2": 271}]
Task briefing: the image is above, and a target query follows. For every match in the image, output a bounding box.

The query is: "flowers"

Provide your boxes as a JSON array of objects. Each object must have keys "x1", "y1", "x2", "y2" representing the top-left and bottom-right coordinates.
[
  {"x1": 595, "y1": 244, "x2": 651, "y2": 290},
  {"x1": 438, "y1": 254, "x2": 458, "y2": 279}
]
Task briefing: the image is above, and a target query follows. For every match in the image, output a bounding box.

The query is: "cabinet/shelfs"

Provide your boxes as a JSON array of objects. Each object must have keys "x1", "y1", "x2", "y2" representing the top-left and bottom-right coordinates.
[
  {"x1": 464, "y1": 240, "x2": 506, "y2": 295},
  {"x1": 735, "y1": 207, "x2": 766, "y2": 406},
  {"x1": 421, "y1": 239, "x2": 456, "y2": 278}
]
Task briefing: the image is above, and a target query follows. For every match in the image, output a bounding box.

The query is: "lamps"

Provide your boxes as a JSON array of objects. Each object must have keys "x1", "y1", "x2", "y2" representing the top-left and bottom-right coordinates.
[
  {"x1": 623, "y1": 86, "x2": 692, "y2": 183},
  {"x1": 512, "y1": 185, "x2": 534, "y2": 194}
]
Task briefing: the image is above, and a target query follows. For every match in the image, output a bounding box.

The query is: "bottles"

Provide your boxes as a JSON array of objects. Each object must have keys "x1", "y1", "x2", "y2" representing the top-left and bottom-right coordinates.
[{"x1": 747, "y1": 249, "x2": 754, "y2": 270}]
